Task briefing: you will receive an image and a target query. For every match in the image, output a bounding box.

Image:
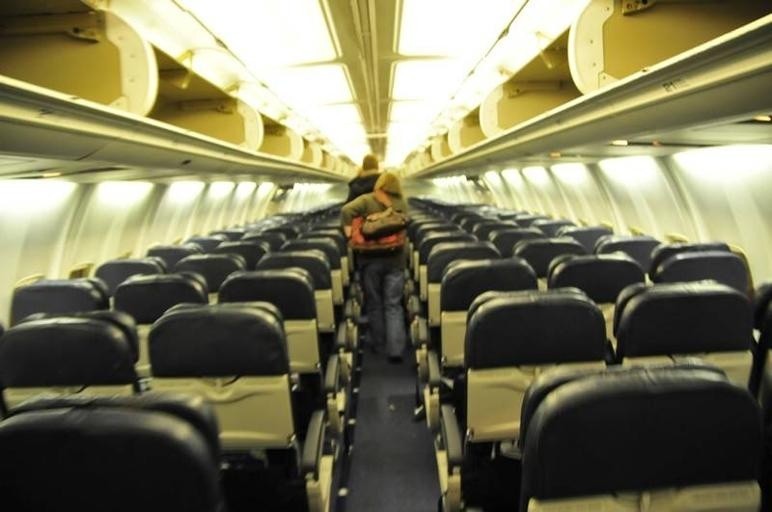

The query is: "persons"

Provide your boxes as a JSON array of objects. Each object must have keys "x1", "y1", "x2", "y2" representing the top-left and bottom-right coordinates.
[
  {"x1": 341, "y1": 172, "x2": 409, "y2": 363},
  {"x1": 344, "y1": 155, "x2": 382, "y2": 205}
]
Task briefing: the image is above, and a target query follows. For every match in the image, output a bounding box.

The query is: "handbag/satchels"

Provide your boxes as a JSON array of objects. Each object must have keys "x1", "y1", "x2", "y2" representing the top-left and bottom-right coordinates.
[
  {"x1": 361, "y1": 208, "x2": 411, "y2": 239},
  {"x1": 351, "y1": 217, "x2": 404, "y2": 255}
]
